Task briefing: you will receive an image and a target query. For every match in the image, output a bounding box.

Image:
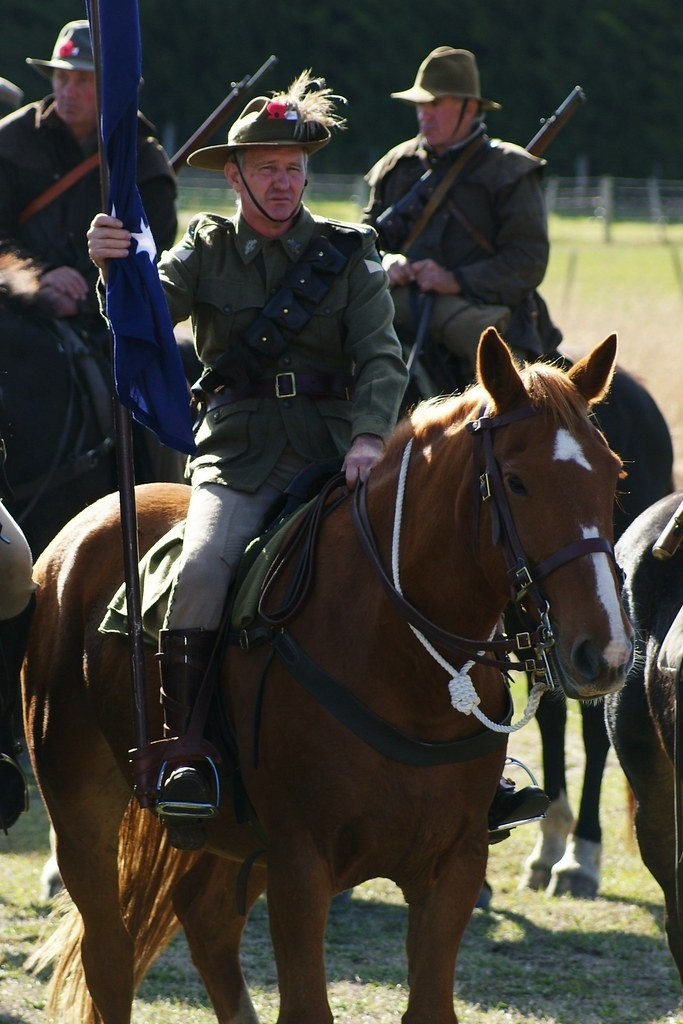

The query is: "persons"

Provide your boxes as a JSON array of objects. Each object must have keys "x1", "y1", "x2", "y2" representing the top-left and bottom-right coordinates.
[
  {"x1": 87, "y1": 102, "x2": 550, "y2": 848},
  {"x1": 356, "y1": 46, "x2": 574, "y2": 417},
  {"x1": 0, "y1": 20, "x2": 178, "y2": 517},
  {"x1": 0, "y1": 500, "x2": 34, "y2": 831}
]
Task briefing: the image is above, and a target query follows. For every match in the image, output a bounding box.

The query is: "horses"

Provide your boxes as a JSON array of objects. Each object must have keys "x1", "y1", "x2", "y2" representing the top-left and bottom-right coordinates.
[{"x1": 0, "y1": 255, "x2": 683, "y2": 1024}]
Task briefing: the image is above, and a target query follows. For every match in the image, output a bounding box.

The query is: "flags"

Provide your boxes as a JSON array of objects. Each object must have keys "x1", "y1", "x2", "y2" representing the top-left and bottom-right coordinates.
[{"x1": 83, "y1": 0, "x2": 199, "y2": 457}]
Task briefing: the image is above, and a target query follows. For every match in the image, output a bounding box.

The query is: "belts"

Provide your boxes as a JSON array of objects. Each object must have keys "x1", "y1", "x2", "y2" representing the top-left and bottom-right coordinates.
[{"x1": 204, "y1": 370, "x2": 354, "y2": 413}]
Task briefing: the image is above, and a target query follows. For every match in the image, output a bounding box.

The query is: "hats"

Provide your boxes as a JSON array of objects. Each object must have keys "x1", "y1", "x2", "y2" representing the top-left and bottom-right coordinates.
[
  {"x1": 391, "y1": 46, "x2": 501, "y2": 111},
  {"x1": 25, "y1": 19, "x2": 144, "y2": 88},
  {"x1": 186, "y1": 67, "x2": 348, "y2": 171},
  {"x1": 0, "y1": 78, "x2": 24, "y2": 106}
]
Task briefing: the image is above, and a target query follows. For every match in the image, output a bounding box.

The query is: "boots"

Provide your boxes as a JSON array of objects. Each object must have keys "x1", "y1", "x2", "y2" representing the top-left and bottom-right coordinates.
[
  {"x1": 0, "y1": 593, "x2": 37, "y2": 829},
  {"x1": 488, "y1": 775, "x2": 550, "y2": 845},
  {"x1": 154, "y1": 626, "x2": 226, "y2": 852}
]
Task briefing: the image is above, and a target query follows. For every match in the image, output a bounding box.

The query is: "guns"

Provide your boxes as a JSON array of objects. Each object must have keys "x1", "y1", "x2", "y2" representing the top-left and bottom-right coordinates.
[
  {"x1": 169, "y1": 55, "x2": 281, "y2": 175},
  {"x1": 525, "y1": 86, "x2": 588, "y2": 158}
]
